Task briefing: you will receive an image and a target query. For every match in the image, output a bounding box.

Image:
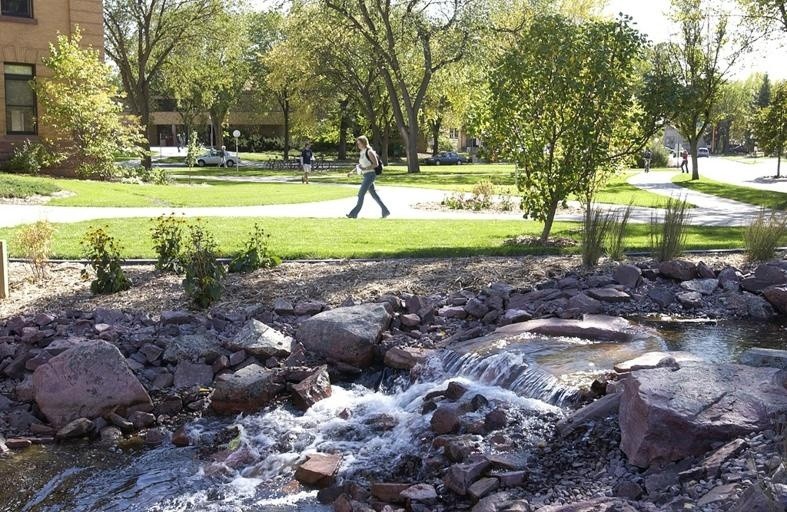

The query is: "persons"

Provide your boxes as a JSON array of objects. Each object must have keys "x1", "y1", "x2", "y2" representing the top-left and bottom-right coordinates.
[
  {"x1": 215, "y1": 146, "x2": 228, "y2": 169},
  {"x1": 180, "y1": 132, "x2": 187, "y2": 149},
  {"x1": 176, "y1": 133, "x2": 181, "y2": 152},
  {"x1": 679, "y1": 150, "x2": 688, "y2": 174},
  {"x1": 300, "y1": 142, "x2": 316, "y2": 184},
  {"x1": 641, "y1": 147, "x2": 653, "y2": 173},
  {"x1": 345, "y1": 136, "x2": 391, "y2": 219}
]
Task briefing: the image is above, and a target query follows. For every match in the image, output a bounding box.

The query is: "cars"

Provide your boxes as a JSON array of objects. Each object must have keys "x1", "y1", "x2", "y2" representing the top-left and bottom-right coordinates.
[
  {"x1": 696, "y1": 148, "x2": 710, "y2": 158},
  {"x1": 184, "y1": 150, "x2": 241, "y2": 167},
  {"x1": 426, "y1": 151, "x2": 467, "y2": 165}
]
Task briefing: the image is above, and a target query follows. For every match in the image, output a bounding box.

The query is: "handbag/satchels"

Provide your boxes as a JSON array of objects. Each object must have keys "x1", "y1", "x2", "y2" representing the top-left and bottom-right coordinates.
[{"x1": 366, "y1": 148, "x2": 383, "y2": 176}]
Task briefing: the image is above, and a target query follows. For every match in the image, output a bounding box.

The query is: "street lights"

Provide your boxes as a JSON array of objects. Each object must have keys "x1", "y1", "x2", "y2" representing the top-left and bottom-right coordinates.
[{"x1": 232, "y1": 130, "x2": 241, "y2": 170}]
